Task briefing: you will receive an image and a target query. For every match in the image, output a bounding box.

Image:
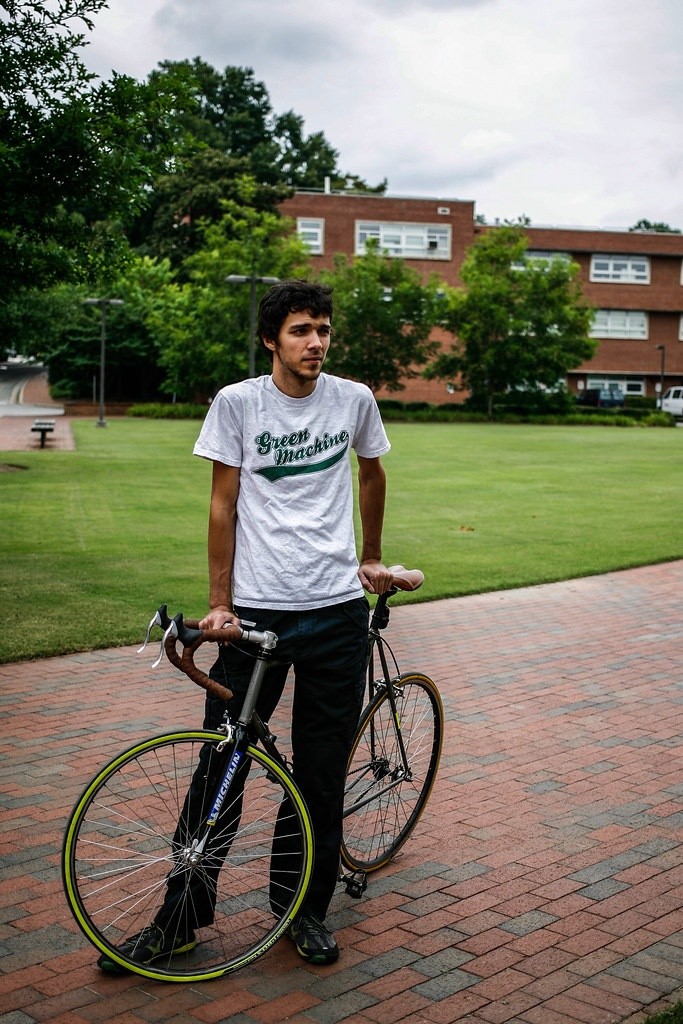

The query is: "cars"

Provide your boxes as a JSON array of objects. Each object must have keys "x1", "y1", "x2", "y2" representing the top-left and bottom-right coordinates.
[{"x1": 576, "y1": 388, "x2": 624, "y2": 410}]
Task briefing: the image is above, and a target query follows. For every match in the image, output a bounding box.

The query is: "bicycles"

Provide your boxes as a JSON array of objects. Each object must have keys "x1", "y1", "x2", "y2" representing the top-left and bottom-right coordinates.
[{"x1": 59, "y1": 564, "x2": 444, "y2": 983}]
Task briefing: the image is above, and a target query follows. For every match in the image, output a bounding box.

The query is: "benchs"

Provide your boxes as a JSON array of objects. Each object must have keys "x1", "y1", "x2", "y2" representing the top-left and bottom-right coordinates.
[{"x1": 32, "y1": 419, "x2": 55, "y2": 448}]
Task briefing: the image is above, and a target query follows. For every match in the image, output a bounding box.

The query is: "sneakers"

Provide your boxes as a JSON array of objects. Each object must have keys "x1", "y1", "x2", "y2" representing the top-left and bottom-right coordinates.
[
  {"x1": 277, "y1": 909, "x2": 343, "y2": 967},
  {"x1": 95, "y1": 919, "x2": 202, "y2": 976}
]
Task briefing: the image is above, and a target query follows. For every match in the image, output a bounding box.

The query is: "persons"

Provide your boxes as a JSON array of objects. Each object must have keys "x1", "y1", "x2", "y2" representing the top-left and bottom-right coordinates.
[{"x1": 94, "y1": 279, "x2": 394, "y2": 976}]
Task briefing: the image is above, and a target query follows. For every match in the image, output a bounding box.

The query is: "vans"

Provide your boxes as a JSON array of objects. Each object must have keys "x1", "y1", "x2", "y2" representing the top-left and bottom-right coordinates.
[{"x1": 657, "y1": 386, "x2": 683, "y2": 422}]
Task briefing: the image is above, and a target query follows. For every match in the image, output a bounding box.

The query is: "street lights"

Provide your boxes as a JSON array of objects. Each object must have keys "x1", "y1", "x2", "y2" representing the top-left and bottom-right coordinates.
[
  {"x1": 655, "y1": 344, "x2": 666, "y2": 410},
  {"x1": 225, "y1": 275, "x2": 283, "y2": 380},
  {"x1": 87, "y1": 298, "x2": 124, "y2": 429}
]
